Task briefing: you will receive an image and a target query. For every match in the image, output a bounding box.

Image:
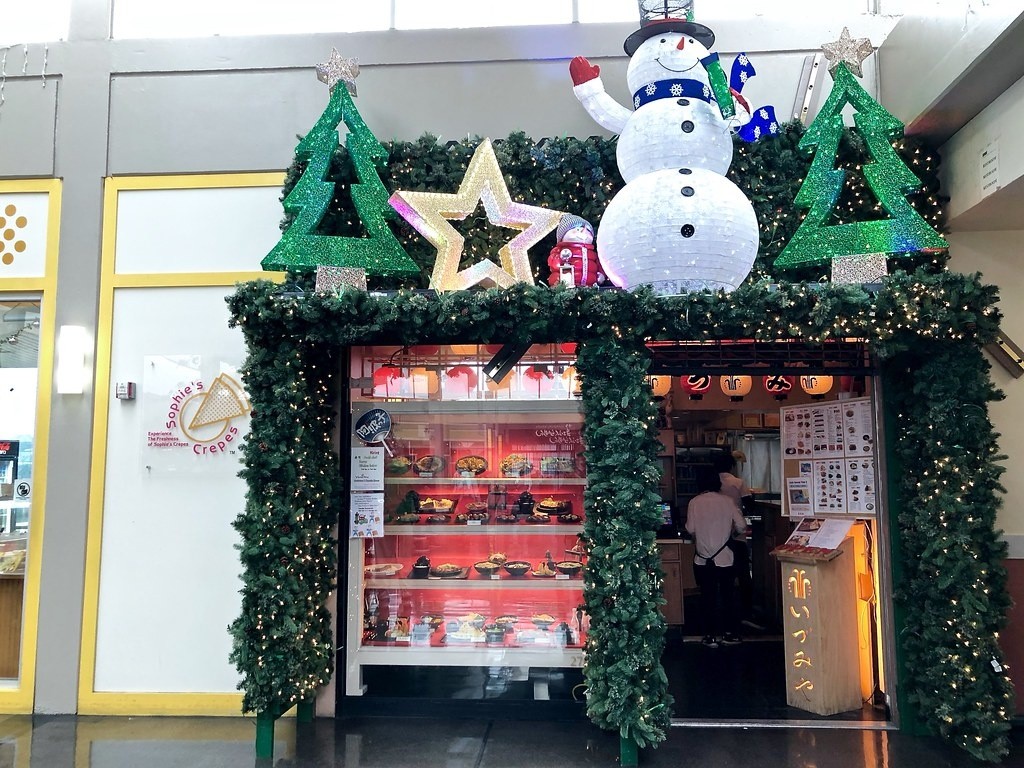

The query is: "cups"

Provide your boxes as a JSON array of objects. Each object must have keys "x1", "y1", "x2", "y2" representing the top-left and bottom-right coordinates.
[{"x1": 413, "y1": 564, "x2": 430, "y2": 577}]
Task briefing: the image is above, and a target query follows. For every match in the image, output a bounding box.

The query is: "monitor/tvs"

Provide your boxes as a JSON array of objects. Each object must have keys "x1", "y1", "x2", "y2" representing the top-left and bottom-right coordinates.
[{"x1": 655, "y1": 500, "x2": 672, "y2": 526}]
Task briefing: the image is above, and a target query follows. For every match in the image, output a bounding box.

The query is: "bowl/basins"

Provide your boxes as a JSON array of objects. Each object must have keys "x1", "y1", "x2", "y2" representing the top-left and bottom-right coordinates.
[
  {"x1": 556, "y1": 561, "x2": 583, "y2": 575},
  {"x1": 474, "y1": 561, "x2": 501, "y2": 576},
  {"x1": 503, "y1": 560, "x2": 532, "y2": 576},
  {"x1": 495, "y1": 615, "x2": 518, "y2": 630},
  {"x1": 484, "y1": 625, "x2": 507, "y2": 641},
  {"x1": 533, "y1": 621, "x2": 553, "y2": 630}
]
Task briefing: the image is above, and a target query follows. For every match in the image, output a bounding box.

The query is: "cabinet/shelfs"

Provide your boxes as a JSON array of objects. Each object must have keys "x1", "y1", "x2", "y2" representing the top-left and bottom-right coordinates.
[
  {"x1": 656, "y1": 543, "x2": 684, "y2": 625},
  {"x1": 673, "y1": 445, "x2": 733, "y2": 508},
  {"x1": 346, "y1": 398, "x2": 591, "y2": 696}
]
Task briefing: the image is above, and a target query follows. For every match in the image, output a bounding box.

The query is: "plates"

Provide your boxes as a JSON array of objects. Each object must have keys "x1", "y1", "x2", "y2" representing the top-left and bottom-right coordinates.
[
  {"x1": 455, "y1": 455, "x2": 488, "y2": 475},
  {"x1": 413, "y1": 455, "x2": 446, "y2": 474},
  {"x1": 431, "y1": 569, "x2": 462, "y2": 577},
  {"x1": 365, "y1": 564, "x2": 404, "y2": 575},
  {"x1": 532, "y1": 570, "x2": 557, "y2": 577}
]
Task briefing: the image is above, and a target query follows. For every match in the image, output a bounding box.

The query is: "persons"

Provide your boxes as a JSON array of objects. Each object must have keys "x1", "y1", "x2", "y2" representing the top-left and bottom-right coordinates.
[{"x1": 684, "y1": 456, "x2": 757, "y2": 648}]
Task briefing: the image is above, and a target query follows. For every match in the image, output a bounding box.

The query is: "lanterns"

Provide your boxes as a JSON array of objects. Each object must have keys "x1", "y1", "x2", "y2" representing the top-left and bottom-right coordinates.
[
  {"x1": 800, "y1": 375, "x2": 834, "y2": 399},
  {"x1": 642, "y1": 375, "x2": 671, "y2": 401},
  {"x1": 762, "y1": 375, "x2": 795, "y2": 400},
  {"x1": 720, "y1": 375, "x2": 752, "y2": 402},
  {"x1": 680, "y1": 375, "x2": 713, "y2": 400}
]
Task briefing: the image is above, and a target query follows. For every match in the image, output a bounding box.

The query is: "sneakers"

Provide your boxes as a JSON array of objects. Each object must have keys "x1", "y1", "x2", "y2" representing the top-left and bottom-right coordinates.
[
  {"x1": 721, "y1": 633, "x2": 742, "y2": 645},
  {"x1": 701, "y1": 636, "x2": 718, "y2": 648}
]
